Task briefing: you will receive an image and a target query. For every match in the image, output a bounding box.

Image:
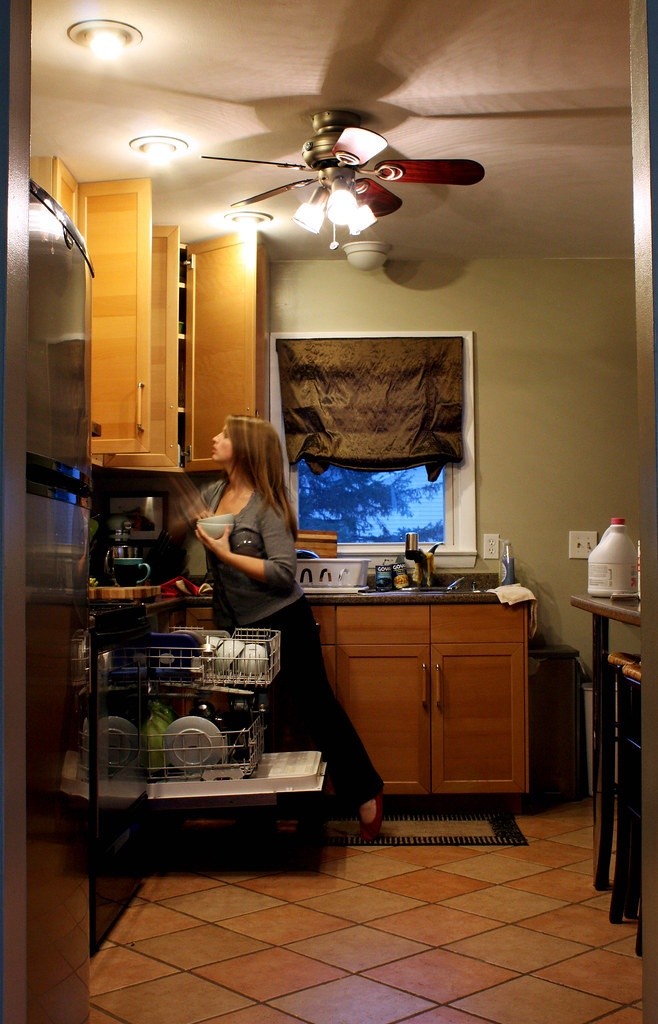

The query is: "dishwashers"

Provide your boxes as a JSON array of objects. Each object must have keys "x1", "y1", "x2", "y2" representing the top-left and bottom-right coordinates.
[{"x1": 90, "y1": 620, "x2": 327, "y2": 956}]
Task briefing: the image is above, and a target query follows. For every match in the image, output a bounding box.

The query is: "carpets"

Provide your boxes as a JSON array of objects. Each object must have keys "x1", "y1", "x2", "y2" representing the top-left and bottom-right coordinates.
[{"x1": 324, "y1": 809, "x2": 529, "y2": 847}]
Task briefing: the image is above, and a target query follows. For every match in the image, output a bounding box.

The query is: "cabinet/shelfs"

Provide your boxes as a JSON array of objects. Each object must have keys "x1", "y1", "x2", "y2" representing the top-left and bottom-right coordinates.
[
  {"x1": 187, "y1": 603, "x2": 529, "y2": 793},
  {"x1": 28, "y1": 153, "x2": 268, "y2": 474}
]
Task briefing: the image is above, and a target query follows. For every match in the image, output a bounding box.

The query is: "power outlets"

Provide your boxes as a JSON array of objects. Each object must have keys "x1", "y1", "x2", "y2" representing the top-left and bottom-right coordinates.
[{"x1": 483, "y1": 534, "x2": 500, "y2": 560}]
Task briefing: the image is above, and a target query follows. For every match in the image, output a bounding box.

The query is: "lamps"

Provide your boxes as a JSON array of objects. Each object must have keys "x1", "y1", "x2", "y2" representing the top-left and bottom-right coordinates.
[
  {"x1": 290, "y1": 178, "x2": 378, "y2": 250},
  {"x1": 340, "y1": 240, "x2": 394, "y2": 272}
]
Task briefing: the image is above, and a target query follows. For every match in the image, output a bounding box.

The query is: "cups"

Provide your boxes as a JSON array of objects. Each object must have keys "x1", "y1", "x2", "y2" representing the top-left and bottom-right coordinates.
[{"x1": 114, "y1": 557, "x2": 150, "y2": 587}]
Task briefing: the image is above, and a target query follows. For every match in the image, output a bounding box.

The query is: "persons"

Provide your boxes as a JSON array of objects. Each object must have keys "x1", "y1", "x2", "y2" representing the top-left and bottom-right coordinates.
[
  {"x1": 169, "y1": 415, "x2": 383, "y2": 842},
  {"x1": 60, "y1": 475, "x2": 90, "y2": 759}
]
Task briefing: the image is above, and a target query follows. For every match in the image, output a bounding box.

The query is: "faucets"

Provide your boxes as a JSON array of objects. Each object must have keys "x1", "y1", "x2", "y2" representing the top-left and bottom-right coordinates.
[{"x1": 405, "y1": 533, "x2": 446, "y2": 588}]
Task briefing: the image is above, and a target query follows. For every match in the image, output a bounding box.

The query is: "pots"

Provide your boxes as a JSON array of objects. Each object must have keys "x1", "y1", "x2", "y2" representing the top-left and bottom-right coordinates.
[{"x1": 170, "y1": 630, "x2": 205, "y2": 646}]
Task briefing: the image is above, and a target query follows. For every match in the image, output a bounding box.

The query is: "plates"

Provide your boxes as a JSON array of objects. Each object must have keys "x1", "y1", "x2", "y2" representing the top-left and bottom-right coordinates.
[
  {"x1": 96, "y1": 716, "x2": 138, "y2": 769},
  {"x1": 165, "y1": 716, "x2": 226, "y2": 771}
]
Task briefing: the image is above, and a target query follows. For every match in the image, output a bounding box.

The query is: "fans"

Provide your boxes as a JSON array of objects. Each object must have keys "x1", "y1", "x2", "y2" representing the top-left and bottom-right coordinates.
[{"x1": 198, "y1": 110, "x2": 485, "y2": 208}]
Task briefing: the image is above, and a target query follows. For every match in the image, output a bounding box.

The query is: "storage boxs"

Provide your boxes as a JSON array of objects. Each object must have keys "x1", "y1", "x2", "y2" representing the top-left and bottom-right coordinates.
[{"x1": 294, "y1": 558, "x2": 372, "y2": 587}]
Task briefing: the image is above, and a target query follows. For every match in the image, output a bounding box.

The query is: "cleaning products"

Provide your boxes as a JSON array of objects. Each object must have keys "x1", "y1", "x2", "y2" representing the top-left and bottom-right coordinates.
[{"x1": 500, "y1": 539, "x2": 515, "y2": 586}]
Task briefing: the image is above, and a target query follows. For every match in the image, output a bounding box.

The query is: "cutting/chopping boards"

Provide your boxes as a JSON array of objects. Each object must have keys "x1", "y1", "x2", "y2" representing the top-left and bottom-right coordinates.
[{"x1": 89, "y1": 586, "x2": 161, "y2": 599}]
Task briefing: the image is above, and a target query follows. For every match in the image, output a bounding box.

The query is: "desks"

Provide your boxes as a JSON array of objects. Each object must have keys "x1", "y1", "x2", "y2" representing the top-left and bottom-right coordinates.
[{"x1": 568, "y1": 594, "x2": 642, "y2": 891}]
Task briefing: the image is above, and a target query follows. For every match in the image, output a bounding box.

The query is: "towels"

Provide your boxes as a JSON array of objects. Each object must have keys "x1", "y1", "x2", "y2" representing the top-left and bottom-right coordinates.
[{"x1": 486, "y1": 582, "x2": 539, "y2": 639}]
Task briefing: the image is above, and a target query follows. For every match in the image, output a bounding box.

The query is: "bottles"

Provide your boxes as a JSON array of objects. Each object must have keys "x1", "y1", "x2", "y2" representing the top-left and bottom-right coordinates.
[
  {"x1": 500, "y1": 540, "x2": 515, "y2": 587},
  {"x1": 587, "y1": 518, "x2": 638, "y2": 597}
]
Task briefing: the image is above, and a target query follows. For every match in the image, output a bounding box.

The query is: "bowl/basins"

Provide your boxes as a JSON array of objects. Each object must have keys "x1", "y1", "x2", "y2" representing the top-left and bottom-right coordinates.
[
  {"x1": 198, "y1": 513, "x2": 234, "y2": 523},
  {"x1": 205, "y1": 635, "x2": 268, "y2": 674},
  {"x1": 197, "y1": 522, "x2": 234, "y2": 539}
]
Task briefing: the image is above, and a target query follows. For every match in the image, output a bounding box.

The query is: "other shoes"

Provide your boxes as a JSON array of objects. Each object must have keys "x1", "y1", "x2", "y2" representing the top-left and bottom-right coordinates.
[{"x1": 360, "y1": 789, "x2": 382, "y2": 840}]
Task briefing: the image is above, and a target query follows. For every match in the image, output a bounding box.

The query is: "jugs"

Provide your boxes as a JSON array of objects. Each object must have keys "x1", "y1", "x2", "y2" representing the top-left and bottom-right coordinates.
[{"x1": 106, "y1": 545, "x2": 138, "y2": 586}]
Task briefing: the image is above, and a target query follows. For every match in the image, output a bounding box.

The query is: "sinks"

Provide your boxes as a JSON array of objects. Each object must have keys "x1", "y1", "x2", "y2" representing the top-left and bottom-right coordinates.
[{"x1": 363, "y1": 587, "x2": 448, "y2": 594}]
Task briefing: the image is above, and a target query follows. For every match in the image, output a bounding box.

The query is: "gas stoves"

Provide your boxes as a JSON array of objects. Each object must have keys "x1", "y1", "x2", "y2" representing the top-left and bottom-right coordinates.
[{"x1": 90, "y1": 605, "x2": 145, "y2": 625}]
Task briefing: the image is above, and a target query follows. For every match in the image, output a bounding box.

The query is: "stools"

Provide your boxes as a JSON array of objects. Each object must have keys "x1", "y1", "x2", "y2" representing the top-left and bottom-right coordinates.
[{"x1": 605, "y1": 651, "x2": 643, "y2": 956}]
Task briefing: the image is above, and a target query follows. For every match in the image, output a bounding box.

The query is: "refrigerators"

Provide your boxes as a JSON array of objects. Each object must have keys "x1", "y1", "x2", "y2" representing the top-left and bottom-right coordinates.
[{"x1": 26, "y1": 186, "x2": 90, "y2": 1023}]
